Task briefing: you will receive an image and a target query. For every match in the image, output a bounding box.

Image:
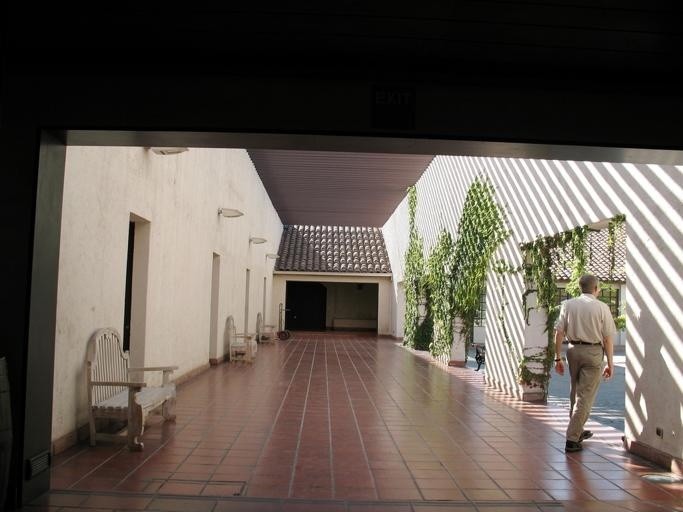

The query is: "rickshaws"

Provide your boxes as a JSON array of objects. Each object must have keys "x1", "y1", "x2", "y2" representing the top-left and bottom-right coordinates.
[{"x1": 278, "y1": 303, "x2": 291, "y2": 340}]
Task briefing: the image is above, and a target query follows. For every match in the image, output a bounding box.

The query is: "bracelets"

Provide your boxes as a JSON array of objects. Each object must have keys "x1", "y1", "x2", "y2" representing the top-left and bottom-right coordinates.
[{"x1": 555, "y1": 359, "x2": 561, "y2": 361}]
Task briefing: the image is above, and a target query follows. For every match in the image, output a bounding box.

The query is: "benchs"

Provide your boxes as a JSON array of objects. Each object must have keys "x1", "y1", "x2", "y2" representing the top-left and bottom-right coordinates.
[
  {"x1": 85, "y1": 326, "x2": 179, "y2": 452},
  {"x1": 226, "y1": 315, "x2": 257, "y2": 362},
  {"x1": 256, "y1": 312, "x2": 277, "y2": 343}
]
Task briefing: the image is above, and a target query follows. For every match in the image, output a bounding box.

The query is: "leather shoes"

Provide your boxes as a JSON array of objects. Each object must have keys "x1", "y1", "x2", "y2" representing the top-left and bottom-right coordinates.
[
  {"x1": 579, "y1": 431, "x2": 593, "y2": 442},
  {"x1": 566, "y1": 440, "x2": 582, "y2": 451}
]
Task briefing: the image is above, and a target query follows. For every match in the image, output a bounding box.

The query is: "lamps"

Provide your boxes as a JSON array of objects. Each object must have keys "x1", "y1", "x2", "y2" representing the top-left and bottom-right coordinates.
[
  {"x1": 265, "y1": 252, "x2": 280, "y2": 259},
  {"x1": 144, "y1": 147, "x2": 189, "y2": 156},
  {"x1": 249, "y1": 237, "x2": 268, "y2": 244},
  {"x1": 217, "y1": 209, "x2": 245, "y2": 218}
]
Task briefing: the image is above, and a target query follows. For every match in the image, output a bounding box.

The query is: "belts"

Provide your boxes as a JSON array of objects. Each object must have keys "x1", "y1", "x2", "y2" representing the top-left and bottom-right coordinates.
[{"x1": 569, "y1": 341, "x2": 600, "y2": 345}]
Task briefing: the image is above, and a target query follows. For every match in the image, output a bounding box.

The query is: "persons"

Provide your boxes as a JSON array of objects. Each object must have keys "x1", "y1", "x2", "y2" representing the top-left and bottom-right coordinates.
[{"x1": 553, "y1": 275, "x2": 618, "y2": 454}]
